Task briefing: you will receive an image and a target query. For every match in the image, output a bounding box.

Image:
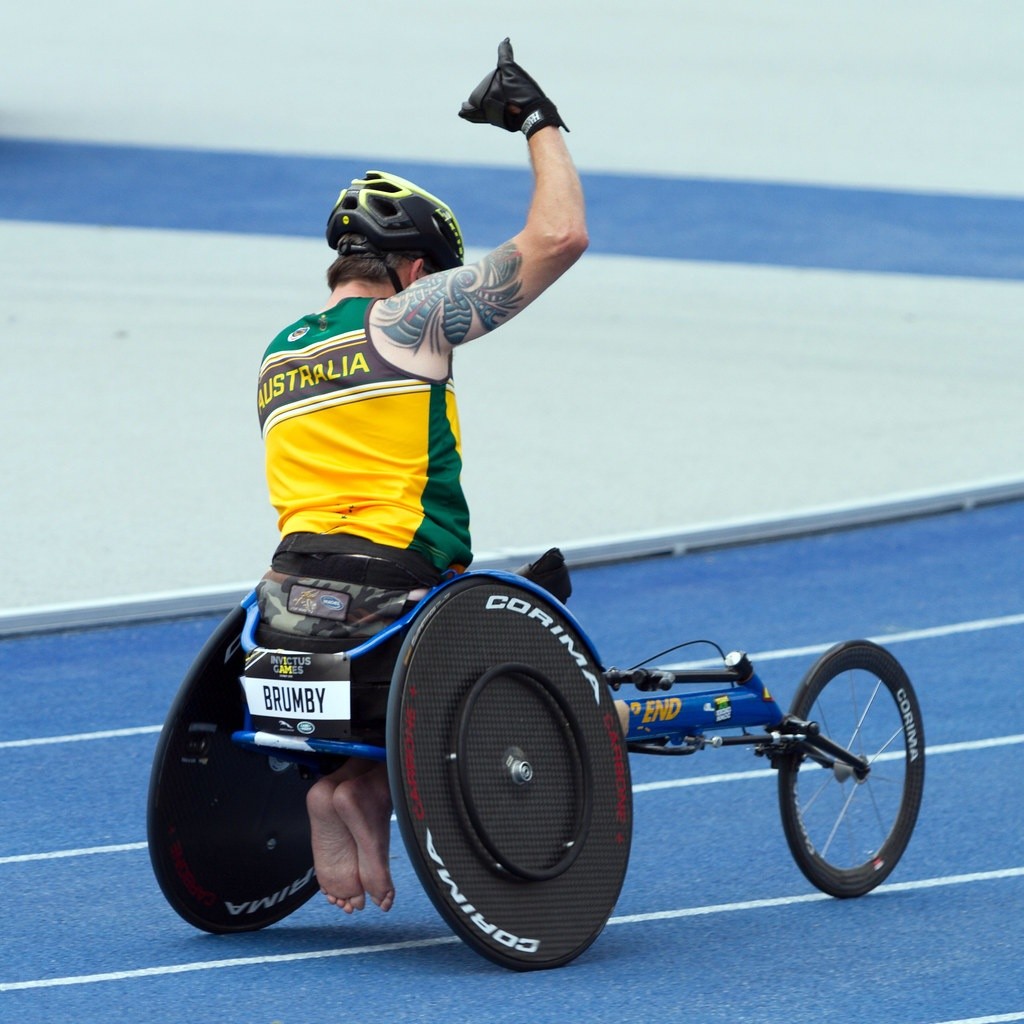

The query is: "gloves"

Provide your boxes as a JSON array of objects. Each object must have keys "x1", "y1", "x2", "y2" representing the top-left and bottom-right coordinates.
[{"x1": 457, "y1": 37, "x2": 570, "y2": 141}]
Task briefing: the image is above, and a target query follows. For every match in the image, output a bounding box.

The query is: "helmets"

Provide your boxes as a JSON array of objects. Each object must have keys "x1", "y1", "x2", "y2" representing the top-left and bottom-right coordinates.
[{"x1": 326, "y1": 169, "x2": 465, "y2": 271}]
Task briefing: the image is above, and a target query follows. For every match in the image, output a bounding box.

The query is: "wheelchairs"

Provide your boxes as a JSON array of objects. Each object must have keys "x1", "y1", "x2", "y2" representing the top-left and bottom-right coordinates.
[{"x1": 140, "y1": 527, "x2": 929, "y2": 973}]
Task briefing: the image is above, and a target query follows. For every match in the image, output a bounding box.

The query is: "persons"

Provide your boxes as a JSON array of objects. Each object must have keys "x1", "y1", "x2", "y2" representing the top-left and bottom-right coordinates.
[{"x1": 256, "y1": 35, "x2": 589, "y2": 915}]
